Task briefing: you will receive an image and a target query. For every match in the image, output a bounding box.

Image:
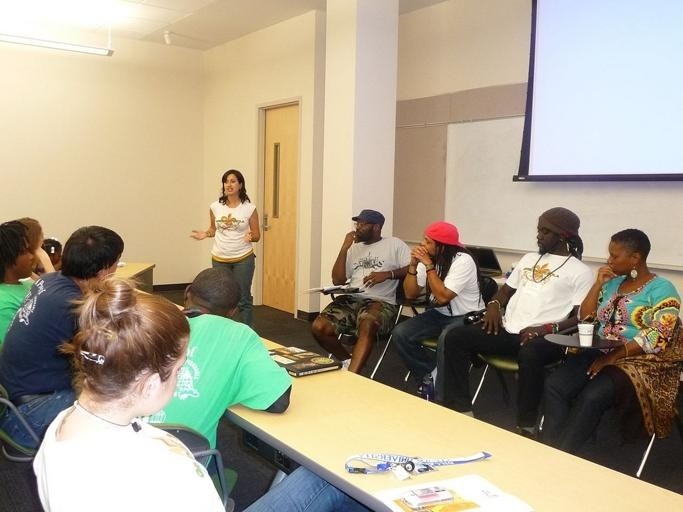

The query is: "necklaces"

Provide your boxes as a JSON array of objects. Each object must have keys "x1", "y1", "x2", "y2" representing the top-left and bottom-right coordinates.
[{"x1": 533, "y1": 253, "x2": 573, "y2": 283}]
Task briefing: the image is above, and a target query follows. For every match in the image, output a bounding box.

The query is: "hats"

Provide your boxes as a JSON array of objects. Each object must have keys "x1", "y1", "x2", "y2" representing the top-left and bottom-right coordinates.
[
  {"x1": 538, "y1": 207, "x2": 580, "y2": 238},
  {"x1": 424, "y1": 222, "x2": 465, "y2": 248},
  {"x1": 352, "y1": 210, "x2": 385, "y2": 225}
]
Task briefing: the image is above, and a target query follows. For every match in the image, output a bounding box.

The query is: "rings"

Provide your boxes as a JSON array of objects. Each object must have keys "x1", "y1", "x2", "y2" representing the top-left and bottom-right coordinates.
[
  {"x1": 535, "y1": 332, "x2": 538, "y2": 336},
  {"x1": 369, "y1": 280, "x2": 373, "y2": 282}
]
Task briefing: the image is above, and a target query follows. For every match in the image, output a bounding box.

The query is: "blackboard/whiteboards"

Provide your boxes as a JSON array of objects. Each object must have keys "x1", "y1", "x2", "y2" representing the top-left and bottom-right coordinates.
[{"x1": 442, "y1": 114, "x2": 683, "y2": 272}]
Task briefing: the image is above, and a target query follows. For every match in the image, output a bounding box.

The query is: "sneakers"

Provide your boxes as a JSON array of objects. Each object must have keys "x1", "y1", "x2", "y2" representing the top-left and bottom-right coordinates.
[{"x1": 341, "y1": 354, "x2": 352, "y2": 370}]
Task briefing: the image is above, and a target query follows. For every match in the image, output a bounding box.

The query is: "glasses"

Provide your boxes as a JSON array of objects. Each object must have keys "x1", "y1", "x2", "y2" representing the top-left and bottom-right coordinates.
[{"x1": 354, "y1": 223, "x2": 368, "y2": 227}]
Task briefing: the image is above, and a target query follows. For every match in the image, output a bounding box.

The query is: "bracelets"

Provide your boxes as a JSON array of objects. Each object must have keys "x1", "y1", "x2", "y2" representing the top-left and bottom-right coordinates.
[
  {"x1": 487, "y1": 300, "x2": 502, "y2": 309},
  {"x1": 426, "y1": 268, "x2": 436, "y2": 273},
  {"x1": 546, "y1": 322, "x2": 559, "y2": 335},
  {"x1": 391, "y1": 270, "x2": 394, "y2": 278},
  {"x1": 408, "y1": 271, "x2": 418, "y2": 276},
  {"x1": 624, "y1": 344, "x2": 630, "y2": 357}
]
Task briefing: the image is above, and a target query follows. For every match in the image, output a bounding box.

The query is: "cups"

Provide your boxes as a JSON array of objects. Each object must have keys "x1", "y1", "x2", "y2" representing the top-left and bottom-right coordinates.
[{"x1": 577, "y1": 323, "x2": 594, "y2": 347}]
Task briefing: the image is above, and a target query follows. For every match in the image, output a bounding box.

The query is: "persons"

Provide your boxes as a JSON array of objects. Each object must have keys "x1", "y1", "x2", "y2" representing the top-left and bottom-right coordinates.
[
  {"x1": 190, "y1": 169, "x2": 260, "y2": 323},
  {"x1": 311, "y1": 209, "x2": 411, "y2": 373},
  {"x1": 444, "y1": 207, "x2": 594, "y2": 440},
  {"x1": 539, "y1": 229, "x2": 683, "y2": 457},
  {"x1": 33, "y1": 280, "x2": 361, "y2": 511},
  {"x1": 391, "y1": 222, "x2": 486, "y2": 400}
]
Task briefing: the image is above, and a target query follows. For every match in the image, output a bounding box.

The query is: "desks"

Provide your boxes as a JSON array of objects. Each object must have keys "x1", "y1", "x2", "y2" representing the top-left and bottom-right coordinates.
[
  {"x1": 157, "y1": 298, "x2": 680, "y2": 512},
  {"x1": 115, "y1": 261, "x2": 156, "y2": 293}
]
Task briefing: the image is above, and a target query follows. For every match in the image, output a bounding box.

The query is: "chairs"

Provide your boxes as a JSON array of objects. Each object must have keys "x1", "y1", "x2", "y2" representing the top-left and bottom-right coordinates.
[
  {"x1": 317, "y1": 260, "x2": 683, "y2": 479},
  {"x1": 1, "y1": 343, "x2": 236, "y2": 512}
]
